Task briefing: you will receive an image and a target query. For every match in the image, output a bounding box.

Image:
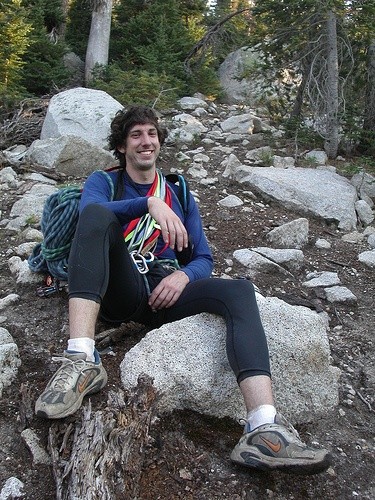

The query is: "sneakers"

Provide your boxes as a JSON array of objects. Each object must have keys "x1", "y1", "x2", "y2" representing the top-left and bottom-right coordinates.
[
  {"x1": 230, "y1": 408, "x2": 331, "y2": 476},
  {"x1": 34, "y1": 344, "x2": 107, "y2": 423}
]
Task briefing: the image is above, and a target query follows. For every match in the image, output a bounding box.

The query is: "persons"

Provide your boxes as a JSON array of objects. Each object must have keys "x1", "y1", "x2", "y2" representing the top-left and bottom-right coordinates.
[{"x1": 36, "y1": 105, "x2": 334, "y2": 477}]
